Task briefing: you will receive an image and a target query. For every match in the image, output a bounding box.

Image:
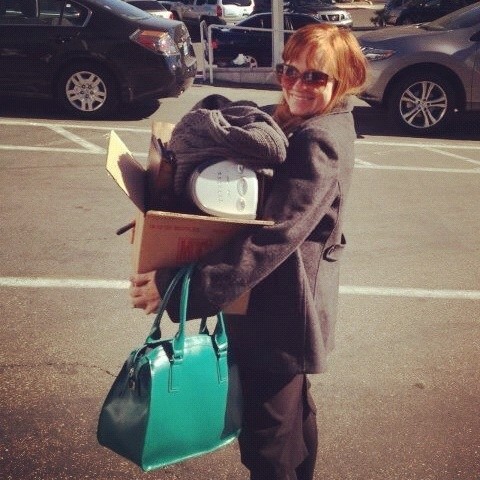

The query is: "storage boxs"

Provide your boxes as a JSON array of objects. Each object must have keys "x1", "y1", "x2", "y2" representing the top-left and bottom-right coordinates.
[{"x1": 104, "y1": 123, "x2": 276, "y2": 316}]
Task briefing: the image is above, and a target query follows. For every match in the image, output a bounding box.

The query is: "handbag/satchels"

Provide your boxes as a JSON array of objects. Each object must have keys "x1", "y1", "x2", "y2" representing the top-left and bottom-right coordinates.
[{"x1": 96, "y1": 264, "x2": 244, "y2": 471}]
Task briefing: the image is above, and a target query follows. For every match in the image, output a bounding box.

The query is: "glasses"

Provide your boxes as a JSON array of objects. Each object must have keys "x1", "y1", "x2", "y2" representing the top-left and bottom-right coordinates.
[{"x1": 276, "y1": 62, "x2": 333, "y2": 94}]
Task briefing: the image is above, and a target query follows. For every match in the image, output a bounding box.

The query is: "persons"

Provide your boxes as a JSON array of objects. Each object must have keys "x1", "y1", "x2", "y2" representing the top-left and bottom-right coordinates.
[{"x1": 128, "y1": 22, "x2": 368, "y2": 480}]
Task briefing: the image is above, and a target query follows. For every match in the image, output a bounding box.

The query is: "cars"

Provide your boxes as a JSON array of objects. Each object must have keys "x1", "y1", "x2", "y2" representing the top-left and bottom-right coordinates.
[
  {"x1": 202, "y1": 12, "x2": 321, "y2": 70},
  {"x1": 282, "y1": 0, "x2": 353, "y2": 34},
  {"x1": 182, "y1": 0, "x2": 255, "y2": 21},
  {"x1": 349, "y1": 0, "x2": 480, "y2": 132},
  {"x1": 0, "y1": 0, "x2": 199, "y2": 119},
  {"x1": 156, "y1": 1, "x2": 206, "y2": 25},
  {"x1": 123, "y1": 0, "x2": 174, "y2": 20}
]
